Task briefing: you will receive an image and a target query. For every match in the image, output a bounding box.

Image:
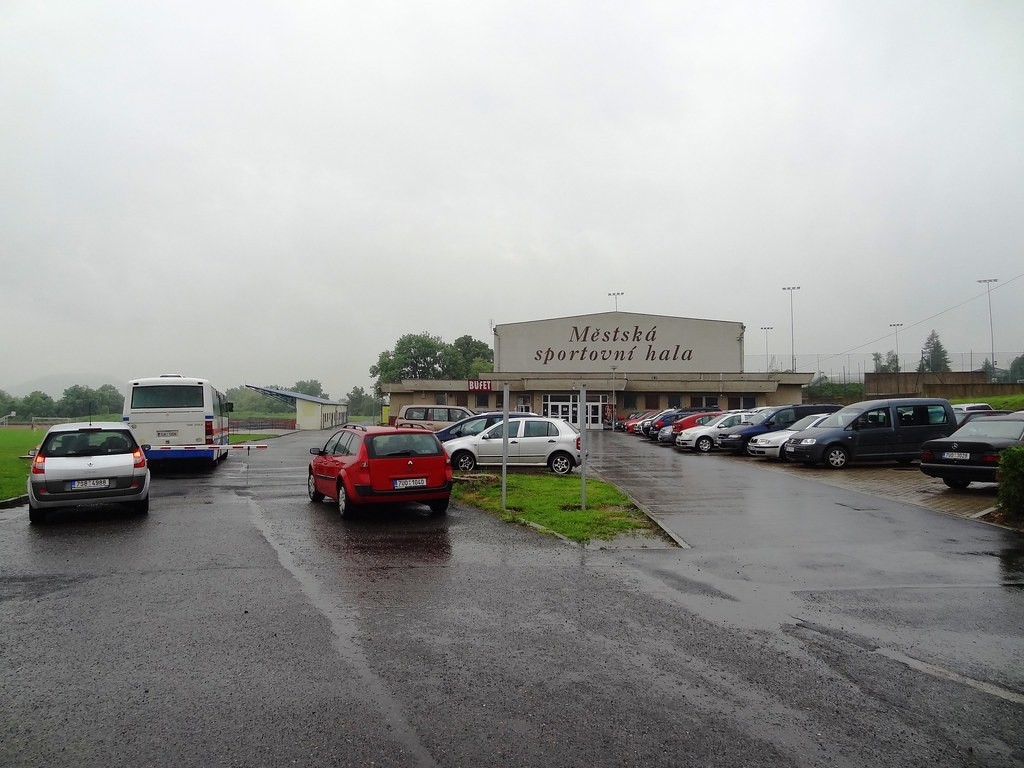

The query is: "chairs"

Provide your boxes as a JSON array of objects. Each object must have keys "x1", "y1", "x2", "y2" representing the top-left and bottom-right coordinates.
[
  {"x1": 897, "y1": 412, "x2": 912, "y2": 426},
  {"x1": 100, "y1": 437, "x2": 127, "y2": 451},
  {"x1": 384, "y1": 437, "x2": 406, "y2": 452},
  {"x1": 56, "y1": 435, "x2": 87, "y2": 454}
]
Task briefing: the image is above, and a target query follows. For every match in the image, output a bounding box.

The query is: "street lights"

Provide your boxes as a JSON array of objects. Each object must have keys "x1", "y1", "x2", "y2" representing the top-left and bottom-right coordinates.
[
  {"x1": 608, "y1": 292, "x2": 624, "y2": 312},
  {"x1": 761, "y1": 327, "x2": 773, "y2": 373},
  {"x1": 782, "y1": 287, "x2": 801, "y2": 373},
  {"x1": 610, "y1": 365, "x2": 619, "y2": 432},
  {"x1": 977, "y1": 279, "x2": 998, "y2": 371},
  {"x1": 890, "y1": 323, "x2": 903, "y2": 398}
]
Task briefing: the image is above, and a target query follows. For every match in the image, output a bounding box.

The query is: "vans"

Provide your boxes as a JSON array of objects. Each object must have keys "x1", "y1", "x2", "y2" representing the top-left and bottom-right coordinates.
[
  {"x1": 784, "y1": 399, "x2": 958, "y2": 469},
  {"x1": 395, "y1": 404, "x2": 476, "y2": 431},
  {"x1": 717, "y1": 403, "x2": 844, "y2": 453}
]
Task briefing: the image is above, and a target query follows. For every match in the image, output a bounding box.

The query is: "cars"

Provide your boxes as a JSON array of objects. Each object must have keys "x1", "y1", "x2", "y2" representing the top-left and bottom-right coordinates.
[
  {"x1": 920, "y1": 414, "x2": 1024, "y2": 488},
  {"x1": 27, "y1": 420, "x2": 152, "y2": 521},
  {"x1": 615, "y1": 406, "x2": 721, "y2": 444},
  {"x1": 902, "y1": 403, "x2": 1024, "y2": 425},
  {"x1": 748, "y1": 411, "x2": 887, "y2": 464},
  {"x1": 675, "y1": 409, "x2": 760, "y2": 453}
]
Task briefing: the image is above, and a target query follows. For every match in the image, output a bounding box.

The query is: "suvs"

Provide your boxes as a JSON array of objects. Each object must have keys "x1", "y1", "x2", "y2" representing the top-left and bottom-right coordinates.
[
  {"x1": 435, "y1": 411, "x2": 540, "y2": 447},
  {"x1": 441, "y1": 418, "x2": 587, "y2": 474},
  {"x1": 307, "y1": 424, "x2": 453, "y2": 518}
]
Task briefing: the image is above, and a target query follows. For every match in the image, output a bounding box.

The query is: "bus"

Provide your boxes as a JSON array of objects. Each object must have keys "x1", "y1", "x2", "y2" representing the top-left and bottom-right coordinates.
[{"x1": 123, "y1": 374, "x2": 233, "y2": 469}]
